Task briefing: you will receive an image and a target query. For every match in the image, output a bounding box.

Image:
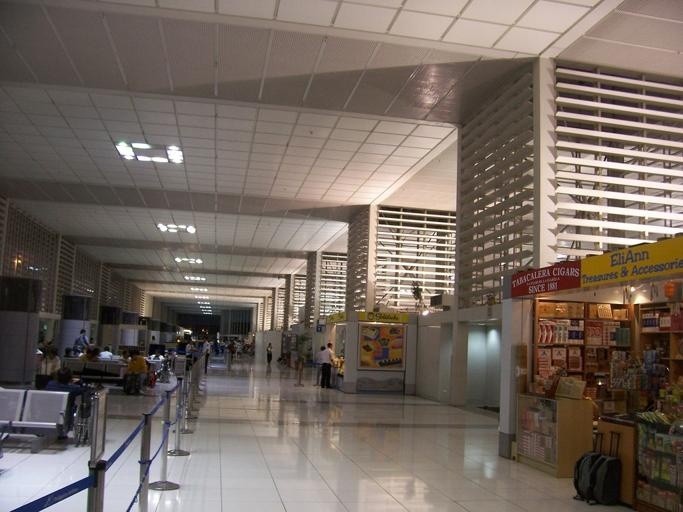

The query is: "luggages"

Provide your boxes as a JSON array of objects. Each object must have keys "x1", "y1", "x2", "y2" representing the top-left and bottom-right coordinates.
[{"x1": 573, "y1": 430, "x2": 624, "y2": 506}]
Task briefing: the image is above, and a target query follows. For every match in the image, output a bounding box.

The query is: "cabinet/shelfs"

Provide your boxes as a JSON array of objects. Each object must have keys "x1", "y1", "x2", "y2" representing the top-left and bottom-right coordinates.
[{"x1": 516, "y1": 298, "x2": 683, "y2": 512}]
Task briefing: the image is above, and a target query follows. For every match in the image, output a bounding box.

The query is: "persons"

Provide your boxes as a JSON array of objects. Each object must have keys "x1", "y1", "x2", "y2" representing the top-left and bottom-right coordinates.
[
  {"x1": 41, "y1": 328, "x2": 113, "y2": 376},
  {"x1": 43, "y1": 366, "x2": 88, "y2": 443},
  {"x1": 148, "y1": 347, "x2": 165, "y2": 363},
  {"x1": 180, "y1": 332, "x2": 245, "y2": 375},
  {"x1": 265, "y1": 341, "x2": 273, "y2": 367},
  {"x1": 124, "y1": 349, "x2": 147, "y2": 396},
  {"x1": 312, "y1": 345, "x2": 325, "y2": 386},
  {"x1": 318, "y1": 342, "x2": 334, "y2": 389}
]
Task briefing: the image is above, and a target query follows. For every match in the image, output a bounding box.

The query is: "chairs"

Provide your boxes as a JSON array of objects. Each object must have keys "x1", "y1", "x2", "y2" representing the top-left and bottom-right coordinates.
[
  {"x1": 60, "y1": 358, "x2": 162, "y2": 389},
  {"x1": 0, "y1": 388, "x2": 71, "y2": 454}
]
